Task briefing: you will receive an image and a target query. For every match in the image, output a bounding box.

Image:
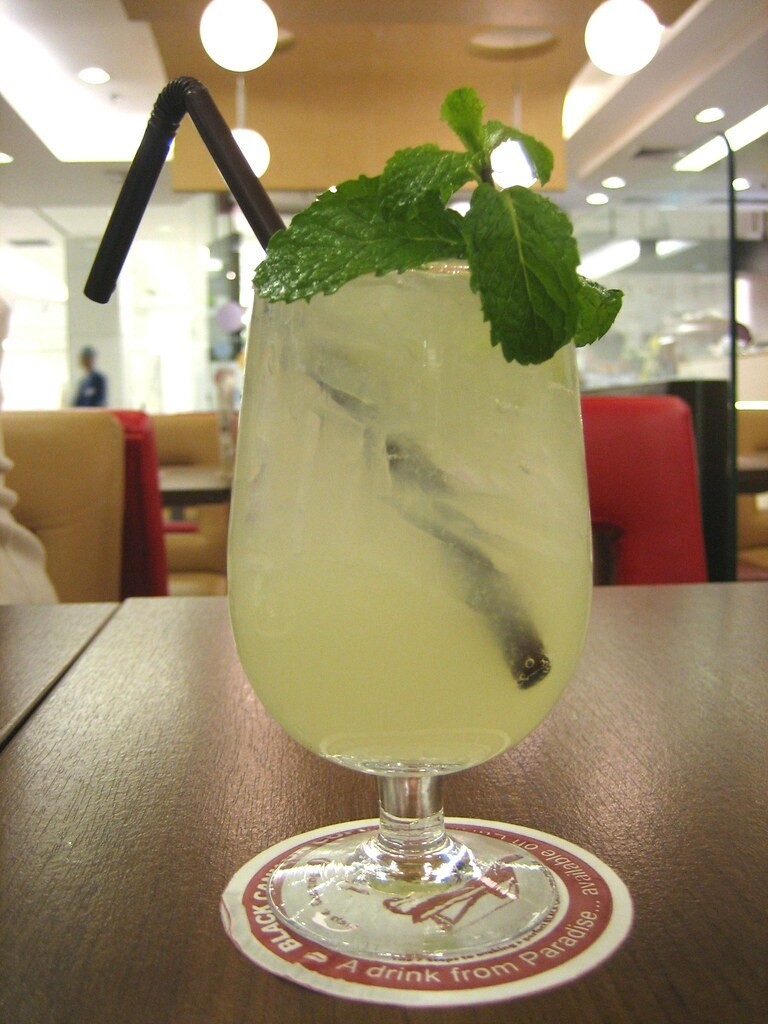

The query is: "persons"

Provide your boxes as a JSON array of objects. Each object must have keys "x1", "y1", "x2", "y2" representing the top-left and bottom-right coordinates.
[{"x1": 70, "y1": 350, "x2": 107, "y2": 406}]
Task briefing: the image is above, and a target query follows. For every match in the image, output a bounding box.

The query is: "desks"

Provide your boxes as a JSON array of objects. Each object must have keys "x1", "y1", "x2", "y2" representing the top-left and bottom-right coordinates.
[
  {"x1": 1, "y1": 582, "x2": 767, "y2": 1024},
  {"x1": 160, "y1": 468, "x2": 230, "y2": 507}
]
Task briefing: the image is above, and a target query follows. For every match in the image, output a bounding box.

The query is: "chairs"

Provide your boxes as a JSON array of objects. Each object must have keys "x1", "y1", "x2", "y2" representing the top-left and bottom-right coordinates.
[{"x1": 1, "y1": 395, "x2": 768, "y2": 606}]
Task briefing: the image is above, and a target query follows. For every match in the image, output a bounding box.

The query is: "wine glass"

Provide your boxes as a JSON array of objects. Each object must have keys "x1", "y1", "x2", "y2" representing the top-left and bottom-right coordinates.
[{"x1": 224, "y1": 257, "x2": 594, "y2": 965}]
